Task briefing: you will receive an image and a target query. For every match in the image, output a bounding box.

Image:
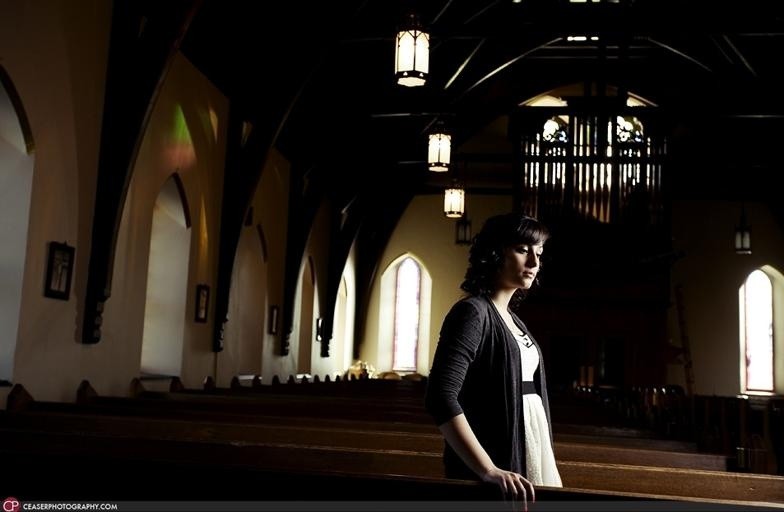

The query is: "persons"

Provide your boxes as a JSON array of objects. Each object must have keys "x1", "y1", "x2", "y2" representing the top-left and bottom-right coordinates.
[{"x1": 423, "y1": 213, "x2": 565, "y2": 511}]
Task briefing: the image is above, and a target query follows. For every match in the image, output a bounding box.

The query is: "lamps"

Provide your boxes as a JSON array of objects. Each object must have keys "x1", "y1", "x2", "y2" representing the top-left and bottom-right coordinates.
[
  {"x1": 732, "y1": 199, "x2": 754, "y2": 257},
  {"x1": 392, "y1": 1, "x2": 468, "y2": 220}
]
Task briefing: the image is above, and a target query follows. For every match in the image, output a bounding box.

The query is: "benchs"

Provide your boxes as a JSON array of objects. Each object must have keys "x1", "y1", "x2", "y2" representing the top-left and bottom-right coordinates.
[{"x1": 5, "y1": 369, "x2": 784, "y2": 512}]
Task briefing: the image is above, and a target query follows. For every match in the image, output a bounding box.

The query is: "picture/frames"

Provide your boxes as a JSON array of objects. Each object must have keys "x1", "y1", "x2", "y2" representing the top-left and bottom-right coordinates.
[
  {"x1": 194, "y1": 283, "x2": 210, "y2": 323},
  {"x1": 41, "y1": 240, "x2": 75, "y2": 303}
]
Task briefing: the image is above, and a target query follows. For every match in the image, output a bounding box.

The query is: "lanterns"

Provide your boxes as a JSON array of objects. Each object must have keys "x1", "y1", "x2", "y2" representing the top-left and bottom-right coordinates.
[
  {"x1": 443, "y1": 177, "x2": 467, "y2": 220},
  {"x1": 426, "y1": 121, "x2": 452, "y2": 173},
  {"x1": 392, "y1": 13, "x2": 433, "y2": 89}
]
